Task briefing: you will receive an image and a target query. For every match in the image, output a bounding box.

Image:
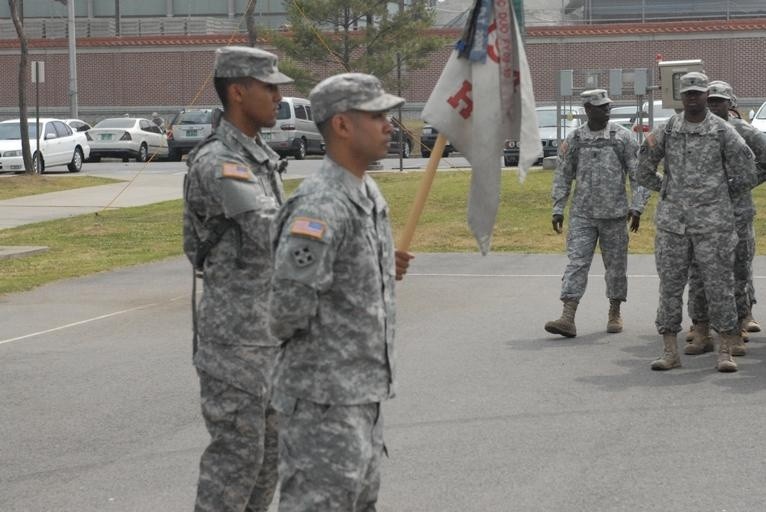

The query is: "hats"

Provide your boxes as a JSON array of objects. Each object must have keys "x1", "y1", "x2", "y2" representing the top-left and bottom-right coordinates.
[
  {"x1": 310, "y1": 73, "x2": 405, "y2": 125},
  {"x1": 708, "y1": 81, "x2": 737, "y2": 108},
  {"x1": 679, "y1": 71, "x2": 709, "y2": 94},
  {"x1": 580, "y1": 89, "x2": 614, "y2": 107},
  {"x1": 215, "y1": 45, "x2": 295, "y2": 85}
]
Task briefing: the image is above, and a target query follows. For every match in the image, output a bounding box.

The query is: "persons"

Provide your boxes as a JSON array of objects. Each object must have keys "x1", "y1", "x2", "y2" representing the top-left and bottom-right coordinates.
[
  {"x1": 635, "y1": 72, "x2": 758, "y2": 373},
  {"x1": 268, "y1": 73, "x2": 405, "y2": 512},
  {"x1": 151, "y1": 112, "x2": 165, "y2": 131},
  {"x1": 545, "y1": 89, "x2": 649, "y2": 337},
  {"x1": 683, "y1": 81, "x2": 766, "y2": 358},
  {"x1": 182, "y1": 45, "x2": 415, "y2": 512}
]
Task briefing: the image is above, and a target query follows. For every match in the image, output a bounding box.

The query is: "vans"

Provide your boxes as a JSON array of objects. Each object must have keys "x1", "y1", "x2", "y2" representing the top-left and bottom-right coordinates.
[
  {"x1": 643, "y1": 101, "x2": 677, "y2": 133},
  {"x1": 258, "y1": 96, "x2": 328, "y2": 159}
]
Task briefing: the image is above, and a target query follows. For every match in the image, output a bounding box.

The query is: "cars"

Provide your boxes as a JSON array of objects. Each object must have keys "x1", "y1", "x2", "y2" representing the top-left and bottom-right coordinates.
[
  {"x1": 63, "y1": 119, "x2": 93, "y2": 134},
  {"x1": 504, "y1": 104, "x2": 588, "y2": 166},
  {"x1": 82, "y1": 117, "x2": 167, "y2": 161},
  {"x1": 421, "y1": 120, "x2": 460, "y2": 158},
  {"x1": 0, "y1": 117, "x2": 90, "y2": 174},
  {"x1": 609, "y1": 106, "x2": 646, "y2": 148},
  {"x1": 387, "y1": 115, "x2": 414, "y2": 158},
  {"x1": 749, "y1": 101, "x2": 766, "y2": 134}
]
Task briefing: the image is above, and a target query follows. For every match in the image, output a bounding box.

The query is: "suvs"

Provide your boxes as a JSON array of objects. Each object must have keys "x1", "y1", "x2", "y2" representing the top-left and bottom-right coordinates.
[{"x1": 168, "y1": 107, "x2": 222, "y2": 162}]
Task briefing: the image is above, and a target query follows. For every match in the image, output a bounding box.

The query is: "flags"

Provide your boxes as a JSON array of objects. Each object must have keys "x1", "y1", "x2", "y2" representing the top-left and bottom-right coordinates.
[{"x1": 418, "y1": 0, "x2": 544, "y2": 255}]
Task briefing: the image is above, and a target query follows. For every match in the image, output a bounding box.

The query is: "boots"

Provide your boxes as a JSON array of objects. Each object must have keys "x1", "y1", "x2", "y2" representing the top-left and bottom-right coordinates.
[
  {"x1": 684, "y1": 324, "x2": 714, "y2": 355},
  {"x1": 651, "y1": 333, "x2": 681, "y2": 370},
  {"x1": 607, "y1": 300, "x2": 623, "y2": 333},
  {"x1": 545, "y1": 299, "x2": 578, "y2": 337},
  {"x1": 731, "y1": 312, "x2": 760, "y2": 356},
  {"x1": 717, "y1": 334, "x2": 738, "y2": 372}
]
